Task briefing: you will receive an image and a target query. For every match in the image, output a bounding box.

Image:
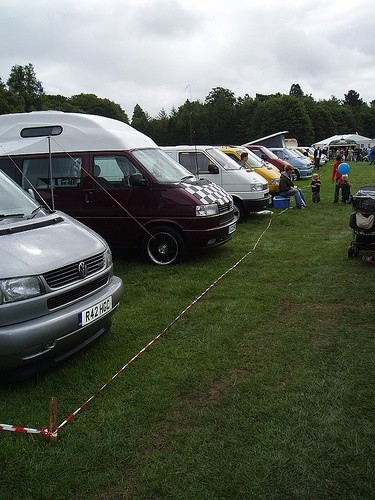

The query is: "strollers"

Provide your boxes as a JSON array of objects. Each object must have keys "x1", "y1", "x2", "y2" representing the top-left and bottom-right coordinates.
[{"x1": 348, "y1": 192, "x2": 375, "y2": 259}]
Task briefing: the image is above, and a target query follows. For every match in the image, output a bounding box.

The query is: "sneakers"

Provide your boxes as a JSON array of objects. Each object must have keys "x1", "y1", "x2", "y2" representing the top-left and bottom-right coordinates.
[{"x1": 360, "y1": 254, "x2": 375, "y2": 268}]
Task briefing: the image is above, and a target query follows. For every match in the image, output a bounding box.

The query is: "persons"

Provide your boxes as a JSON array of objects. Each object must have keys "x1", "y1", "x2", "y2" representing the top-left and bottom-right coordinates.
[
  {"x1": 303, "y1": 149, "x2": 309, "y2": 157},
  {"x1": 369, "y1": 146, "x2": 375, "y2": 165},
  {"x1": 331, "y1": 155, "x2": 348, "y2": 204},
  {"x1": 314, "y1": 146, "x2": 321, "y2": 170},
  {"x1": 321, "y1": 147, "x2": 368, "y2": 162},
  {"x1": 341, "y1": 175, "x2": 351, "y2": 204},
  {"x1": 237, "y1": 153, "x2": 248, "y2": 168},
  {"x1": 311, "y1": 173, "x2": 322, "y2": 203},
  {"x1": 279, "y1": 165, "x2": 310, "y2": 207}
]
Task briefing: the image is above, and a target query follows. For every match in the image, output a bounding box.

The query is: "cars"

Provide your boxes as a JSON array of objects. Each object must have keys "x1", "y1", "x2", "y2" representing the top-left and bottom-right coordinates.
[{"x1": 0, "y1": 167, "x2": 124, "y2": 375}]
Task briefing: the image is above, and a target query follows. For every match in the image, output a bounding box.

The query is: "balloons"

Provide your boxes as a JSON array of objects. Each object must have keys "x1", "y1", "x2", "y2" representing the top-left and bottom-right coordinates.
[{"x1": 338, "y1": 163, "x2": 350, "y2": 174}]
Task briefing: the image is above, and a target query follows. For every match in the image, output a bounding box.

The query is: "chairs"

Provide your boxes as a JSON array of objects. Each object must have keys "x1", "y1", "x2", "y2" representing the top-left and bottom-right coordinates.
[{"x1": 94, "y1": 165, "x2": 111, "y2": 187}]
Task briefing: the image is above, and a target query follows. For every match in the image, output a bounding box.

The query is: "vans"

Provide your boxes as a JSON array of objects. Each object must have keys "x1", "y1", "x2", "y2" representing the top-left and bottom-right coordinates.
[
  {"x1": 220, "y1": 144, "x2": 316, "y2": 195},
  {"x1": 0, "y1": 112, "x2": 240, "y2": 267},
  {"x1": 75, "y1": 146, "x2": 270, "y2": 224}
]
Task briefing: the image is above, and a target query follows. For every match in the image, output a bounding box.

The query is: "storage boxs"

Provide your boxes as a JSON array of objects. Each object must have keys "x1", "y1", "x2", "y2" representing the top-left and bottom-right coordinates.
[{"x1": 273, "y1": 195, "x2": 290, "y2": 209}]
